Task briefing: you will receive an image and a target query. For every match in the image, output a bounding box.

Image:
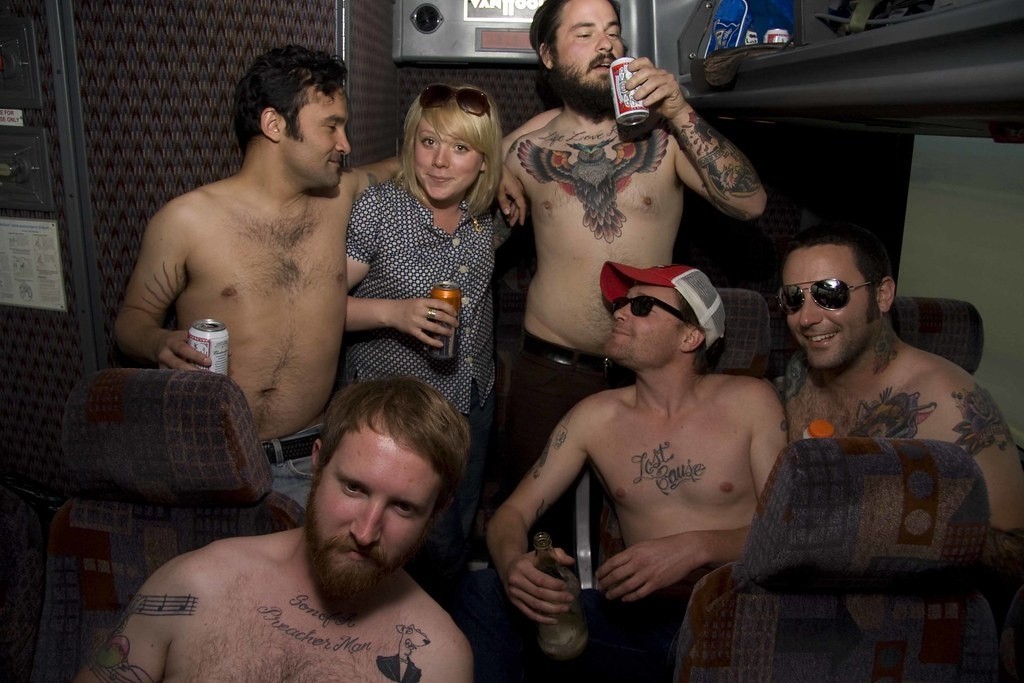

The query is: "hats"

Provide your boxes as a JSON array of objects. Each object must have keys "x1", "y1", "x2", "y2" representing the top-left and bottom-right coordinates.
[{"x1": 600, "y1": 261, "x2": 726, "y2": 350}]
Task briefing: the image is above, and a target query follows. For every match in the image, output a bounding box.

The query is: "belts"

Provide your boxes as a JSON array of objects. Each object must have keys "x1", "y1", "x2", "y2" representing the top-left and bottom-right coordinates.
[
  {"x1": 523, "y1": 332, "x2": 625, "y2": 372},
  {"x1": 259, "y1": 433, "x2": 321, "y2": 464}
]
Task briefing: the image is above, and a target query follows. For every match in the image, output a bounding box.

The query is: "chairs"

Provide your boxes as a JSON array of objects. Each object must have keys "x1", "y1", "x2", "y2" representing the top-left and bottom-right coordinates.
[
  {"x1": 575, "y1": 285, "x2": 1024, "y2": 683},
  {"x1": 0, "y1": 367, "x2": 310, "y2": 683}
]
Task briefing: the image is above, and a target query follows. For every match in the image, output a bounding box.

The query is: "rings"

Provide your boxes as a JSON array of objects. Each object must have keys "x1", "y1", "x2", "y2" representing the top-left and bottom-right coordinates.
[{"x1": 427, "y1": 308, "x2": 437, "y2": 319}]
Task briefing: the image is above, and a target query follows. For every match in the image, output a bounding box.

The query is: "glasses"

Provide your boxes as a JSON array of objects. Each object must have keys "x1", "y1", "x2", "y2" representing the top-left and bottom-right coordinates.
[
  {"x1": 419, "y1": 84, "x2": 490, "y2": 120},
  {"x1": 775, "y1": 279, "x2": 876, "y2": 314},
  {"x1": 610, "y1": 296, "x2": 689, "y2": 323}
]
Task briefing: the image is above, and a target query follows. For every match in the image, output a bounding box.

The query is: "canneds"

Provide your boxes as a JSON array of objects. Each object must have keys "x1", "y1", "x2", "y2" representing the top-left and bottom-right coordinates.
[
  {"x1": 609, "y1": 56, "x2": 649, "y2": 125},
  {"x1": 430, "y1": 281, "x2": 459, "y2": 361},
  {"x1": 188, "y1": 318, "x2": 228, "y2": 376},
  {"x1": 763, "y1": 28, "x2": 789, "y2": 44}
]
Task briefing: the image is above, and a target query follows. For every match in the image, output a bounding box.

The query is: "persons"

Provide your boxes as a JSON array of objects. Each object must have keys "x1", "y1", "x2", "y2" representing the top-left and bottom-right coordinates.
[
  {"x1": 491, "y1": 0, "x2": 766, "y2": 578},
  {"x1": 487, "y1": 260, "x2": 787, "y2": 683},
  {"x1": 115, "y1": 43, "x2": 526, "y2": 527},
  {"x1": 73, "y1": 375, "x2": 474, "y2": 683},
  {"x1": 339, "y1": 82, "x2": 565, "y2": 683},
  {"x1": 776, "y1": 223, "x2": 1024, "y2": 575}
]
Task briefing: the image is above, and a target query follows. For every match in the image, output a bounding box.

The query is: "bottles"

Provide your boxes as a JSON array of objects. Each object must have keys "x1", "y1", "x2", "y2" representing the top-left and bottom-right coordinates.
[
  {"x1": 531, "y1": 532, "x2": 588, "y2": 660},
  {"x1": 803, "y1": 419, "x2": 834, "y2": 440}
]
